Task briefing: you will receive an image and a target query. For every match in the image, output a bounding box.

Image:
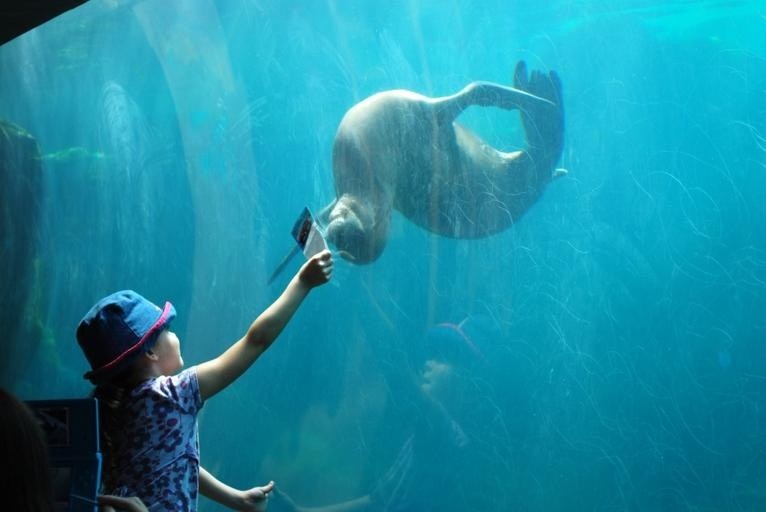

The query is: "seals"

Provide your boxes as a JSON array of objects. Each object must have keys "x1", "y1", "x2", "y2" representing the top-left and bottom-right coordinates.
[{"x1": 267, "y1": 59, "x2": 569, "y2": 287}]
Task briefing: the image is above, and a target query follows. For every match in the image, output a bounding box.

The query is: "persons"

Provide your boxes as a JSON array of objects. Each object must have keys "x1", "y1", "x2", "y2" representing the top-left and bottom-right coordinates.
[
  {"x1": 271, "y1": 313, "x2": 518, "y2": 512},
  {"x1": 76, "y1": 248, "x2": 333, "y2": 512},
  {"x1": 0, "y1": 390, "x2": 147, "y2": 512}
]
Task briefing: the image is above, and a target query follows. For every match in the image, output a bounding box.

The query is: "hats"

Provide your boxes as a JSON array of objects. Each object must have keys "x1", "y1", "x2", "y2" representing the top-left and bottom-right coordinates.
[{"x1": 76, "y1": 290, "x2": 175, "y2": 378}]
